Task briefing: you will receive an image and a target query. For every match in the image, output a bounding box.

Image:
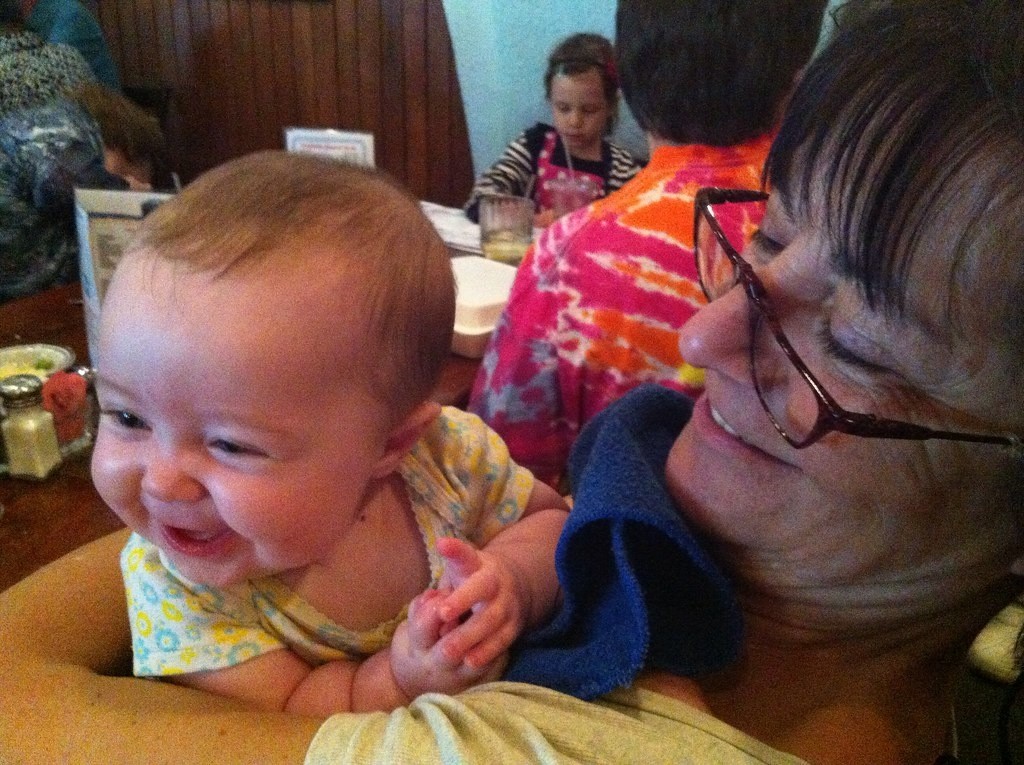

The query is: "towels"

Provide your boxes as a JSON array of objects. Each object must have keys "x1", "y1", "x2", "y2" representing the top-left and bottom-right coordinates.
[{"x1": 498, "y1": 380, "x2": 752, "y2": 701}]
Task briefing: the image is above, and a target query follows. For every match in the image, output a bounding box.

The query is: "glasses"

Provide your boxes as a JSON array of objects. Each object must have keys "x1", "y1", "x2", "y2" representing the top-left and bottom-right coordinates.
[{"x1": 693, "y1": 187, "x2": 1018, "y2": 449}]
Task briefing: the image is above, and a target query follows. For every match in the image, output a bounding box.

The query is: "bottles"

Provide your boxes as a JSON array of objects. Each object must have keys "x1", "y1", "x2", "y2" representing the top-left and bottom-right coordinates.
[
  {"x1": 0, "y1": 375, "x2": 63, "y2": 481},
  {"x1": 43, "y1": 369, "x2": 92, "y2": 457}
]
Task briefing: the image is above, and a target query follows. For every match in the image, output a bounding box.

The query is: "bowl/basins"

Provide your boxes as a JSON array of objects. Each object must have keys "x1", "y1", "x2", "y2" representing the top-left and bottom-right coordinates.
[
  {"x1": 440, "y1": 257, "x2": 520, "y2": 359},
  {"x1": 1, "y1": 344, "x2": 76, "y2": 380}
]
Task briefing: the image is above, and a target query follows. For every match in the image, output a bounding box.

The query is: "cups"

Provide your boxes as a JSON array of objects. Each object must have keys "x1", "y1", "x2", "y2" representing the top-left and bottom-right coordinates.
[
  {"x1": 544, "y1": 178, "x2": 598, "y2": 222},
  {"x1": 478, "y1": 195, "x2": 535, "y2": 263}
]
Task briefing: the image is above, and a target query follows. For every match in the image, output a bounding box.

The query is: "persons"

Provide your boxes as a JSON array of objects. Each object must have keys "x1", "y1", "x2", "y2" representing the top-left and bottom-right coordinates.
[
  {"x1": 0, "y1": 0, "x2": 1024, "y2": 765},
  {"x1": 88, "y1": 155, "x2": 573, "y2": 719},
  {"x1": 463, "y1": 0, "x2": 828, "y2": 497},
  {"x1": 0, "y1": 0, "x2": 165, "y2": 302},
  {"x1": 464, "y1": 32, "x2": 641, "y2": 229}
]
